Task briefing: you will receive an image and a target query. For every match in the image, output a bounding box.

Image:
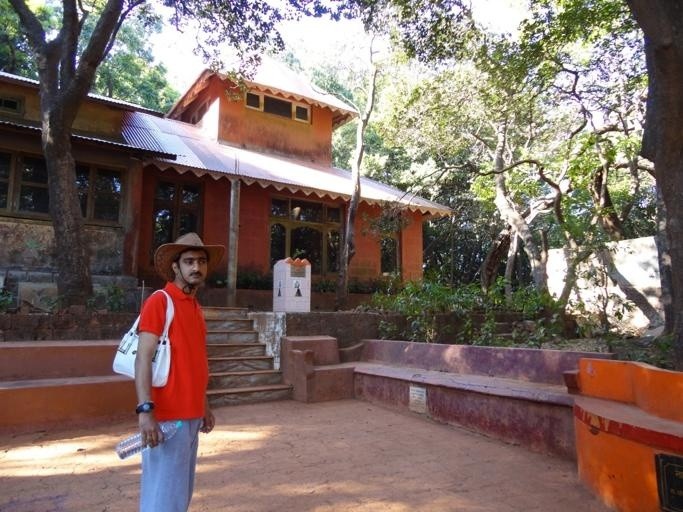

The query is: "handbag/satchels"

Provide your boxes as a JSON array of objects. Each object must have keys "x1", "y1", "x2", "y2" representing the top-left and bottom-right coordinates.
[{"x1": 112, "y1": 330, "x2": 172, "y2": 388}]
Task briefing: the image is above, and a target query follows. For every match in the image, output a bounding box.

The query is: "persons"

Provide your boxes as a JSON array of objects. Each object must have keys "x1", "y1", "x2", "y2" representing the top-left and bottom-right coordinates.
[{"x1": 134, "y1": 232, "x2": 226, "y2": 511}]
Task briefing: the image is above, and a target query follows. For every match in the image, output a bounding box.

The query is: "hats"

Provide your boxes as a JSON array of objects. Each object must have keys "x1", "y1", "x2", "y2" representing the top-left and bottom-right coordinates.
[{"x1": 153, "y1": 232, "x2": 226, "y2": 283}]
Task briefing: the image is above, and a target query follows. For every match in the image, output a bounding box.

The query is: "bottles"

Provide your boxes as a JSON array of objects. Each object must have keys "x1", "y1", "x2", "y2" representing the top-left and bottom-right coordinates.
[{"x1": 116, "y1": 419, "x2": 184, "y2": 460}]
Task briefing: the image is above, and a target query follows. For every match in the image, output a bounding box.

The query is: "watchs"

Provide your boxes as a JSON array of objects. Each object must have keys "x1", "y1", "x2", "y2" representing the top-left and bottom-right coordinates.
[{"x1": 134, "y1": 399, "x2": 152, "y2": 413}]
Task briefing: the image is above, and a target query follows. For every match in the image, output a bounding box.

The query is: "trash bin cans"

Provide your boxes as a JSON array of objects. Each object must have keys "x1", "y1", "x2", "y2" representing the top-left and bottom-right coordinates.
[{"x1": 273, "y1": 257, "x2": 311, "y2": 313}]
